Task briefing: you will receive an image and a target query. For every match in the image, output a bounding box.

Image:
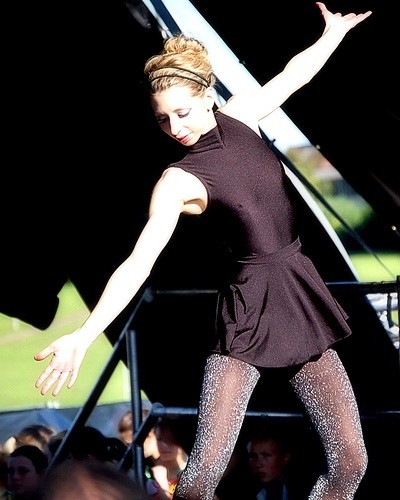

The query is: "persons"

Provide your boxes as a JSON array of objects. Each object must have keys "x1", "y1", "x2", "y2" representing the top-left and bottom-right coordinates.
[
  {"x1": 33, "y1": 2, "x2": 372, "y2": 500},
  {"x1": 0, "y1": 413, "x2": 294, "y2": 500}
]
